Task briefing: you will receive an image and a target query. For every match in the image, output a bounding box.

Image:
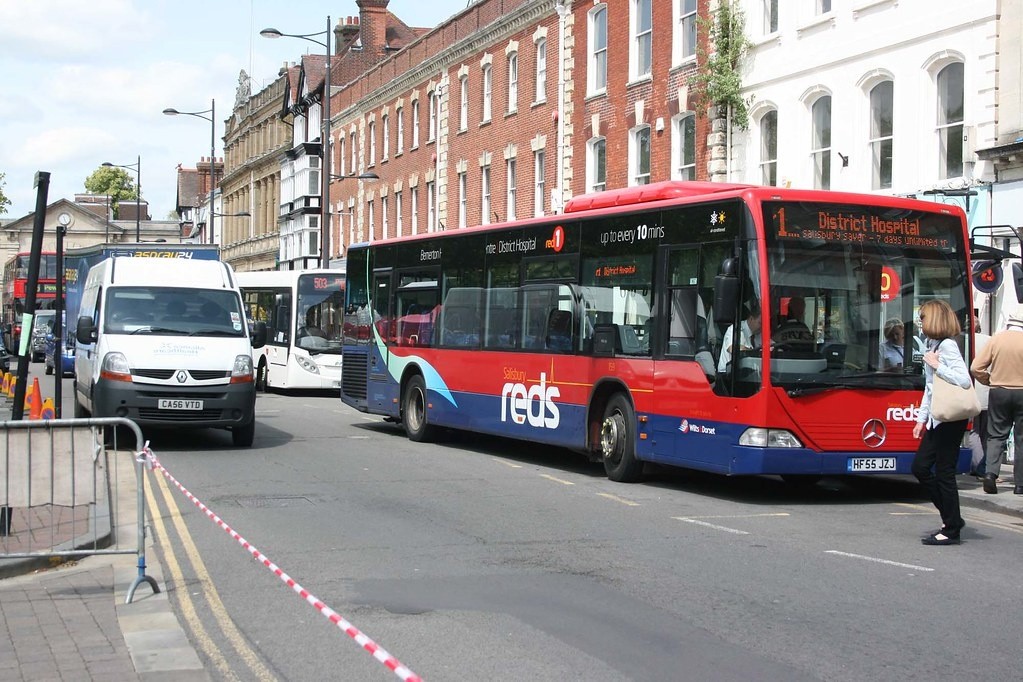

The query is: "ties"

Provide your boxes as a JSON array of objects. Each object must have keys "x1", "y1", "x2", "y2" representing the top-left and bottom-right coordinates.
[{"x1": 751, "y1": 335, "x2": 755, "y2": 349}]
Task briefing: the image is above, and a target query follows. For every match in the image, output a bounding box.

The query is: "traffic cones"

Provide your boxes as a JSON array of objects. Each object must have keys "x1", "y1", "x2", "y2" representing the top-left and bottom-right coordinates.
[
  {"x1": 24, "y1": 384, "x2": 33, "y2": 411},
  {"x1": 5, "y1": 376, "x2": 16, "y2": 403},
  {"x1": 0, "y1": 370, "x2": 12, "y2": 398},
  {"x1": 30, "y1": 377, "x2": 43, "y2": 420},
  {"x1": 39, "y1": 398, "x2": 55, "y2": 420}
]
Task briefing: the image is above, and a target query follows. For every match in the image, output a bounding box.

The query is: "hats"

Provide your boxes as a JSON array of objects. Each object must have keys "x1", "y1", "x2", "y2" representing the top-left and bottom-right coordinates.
[{"x1": 1007, "y1": 312, "x2": 1023, "y2": 327}]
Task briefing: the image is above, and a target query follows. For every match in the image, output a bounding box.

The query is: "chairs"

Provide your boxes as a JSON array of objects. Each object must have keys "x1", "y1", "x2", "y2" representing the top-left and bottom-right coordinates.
[
  {"x1": 402, "y1": 304, "x2": 574, "y2": 354},
  {"x1": 162, "y1": 301, "x2": 188, "y2": 322},
  {"x1": 588, "y1": 324, "x2": 650, "y2": 359},
  {"x1": 194, "y1": 302, "x2": 221, "y2": 325}
]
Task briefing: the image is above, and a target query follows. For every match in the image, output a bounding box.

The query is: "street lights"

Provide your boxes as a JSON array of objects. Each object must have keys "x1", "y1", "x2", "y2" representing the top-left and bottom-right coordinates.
[
  {"x1": 260, "y1": 16, "x2": 379, "y2": 269},
  {"x1": 102, "y1": 155, "x2": 165, "y2": 242},
  {"x1": 163, "y1": 99, "x2": 252, "y2": 244}
]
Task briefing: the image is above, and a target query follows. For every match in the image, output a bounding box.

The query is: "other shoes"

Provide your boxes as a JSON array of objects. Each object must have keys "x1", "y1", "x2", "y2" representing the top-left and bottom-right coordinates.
[
  {"x1": 1014, "y1": 485, "x2": 1023, "y2": 494},
  {"x1": 931, "y1": 517, "x2": 965, "y2": 536},
  {"x1": 984, "y1": 473, "x2": 997, "y2": 494},
  {"x1": 921, "y1": 532, "x2": 961, "y2": 545}
]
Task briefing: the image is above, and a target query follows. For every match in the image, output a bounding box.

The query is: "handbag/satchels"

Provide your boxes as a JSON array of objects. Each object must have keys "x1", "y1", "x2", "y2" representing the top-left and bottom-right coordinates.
[{"x1": 932, "y1": 337, "x2": 982, "y2": 422}]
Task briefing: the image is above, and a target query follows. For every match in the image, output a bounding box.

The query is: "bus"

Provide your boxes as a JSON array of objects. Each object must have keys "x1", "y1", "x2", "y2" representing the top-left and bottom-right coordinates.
[
  {"x1": 341, "y1": 180, "x2": 1023, "y2": 483},
  {"x1": 234, "y1": 269, "x2": 346, "y2": 392},
  {"x1": 3, "y1": 252, "x2": 66, "y2": 356}
]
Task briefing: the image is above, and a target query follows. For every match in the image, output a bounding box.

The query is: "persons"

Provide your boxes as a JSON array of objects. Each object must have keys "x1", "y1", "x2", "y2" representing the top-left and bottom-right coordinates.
[
  {"x1": 357, "y1": 295, "x2": 382, "y2": 325},
  {"x1": 965, "y1": 314, "x2": 992, "y2": 460},
  {"x1": 250, "y1": 305, "x2": 269, "y2": 321},
  {"x1": 970, "y1": 313, "x2": 1023, "y2": 495},
  {"x1": 880, "y1": 318, "x2": 937, "y2": 376},
  {"x1": 0, "y1": 322, "x2": 7, "y2": 351},
  {"x1": 322, "y1": 302, "x2": 344, "y2": 340},
  {"x1": 717, "y1": 304, "x2": 783, "y2": 395},
  {"x1": 112, "y1": 299, "x2": 148, "y2": 325},
  {"x1": 565, "y1": 301, "x2": 595, "y2": 350},
  {"x1": 816, "y1": 320, "x2": 841, "y2": 343},
  {"x1": 772, "y1": 297, "x2": 816, "y2": 349},
  {"x1": 913, "y1": 301, "x2": 971, "y2": 545}
]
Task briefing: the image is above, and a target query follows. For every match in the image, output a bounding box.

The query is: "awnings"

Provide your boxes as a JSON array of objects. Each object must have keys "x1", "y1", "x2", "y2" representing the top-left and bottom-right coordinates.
[{"x1": 188, "y1": 223, "x2": 202, "y2": 237}]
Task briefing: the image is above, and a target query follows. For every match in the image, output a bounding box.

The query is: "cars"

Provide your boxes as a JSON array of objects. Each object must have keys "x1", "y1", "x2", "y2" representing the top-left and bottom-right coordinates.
[
  {"x1": 29, "y1": 310, "x2": 66, "y2": 363},
  {"x1": 44, "y1": 316, "x2": 75, "y2": 377},
  {"x1": 0, "y1": 347, "x2": 10, "y2": 373}
]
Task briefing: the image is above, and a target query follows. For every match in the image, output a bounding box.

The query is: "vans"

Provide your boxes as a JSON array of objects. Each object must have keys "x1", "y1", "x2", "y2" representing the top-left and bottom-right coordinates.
[
  {"x1": 452, "y1": 325, "x2": 455, "y2": 327},
  {"x1": 435, "y1": 283, "x2": 649, "y2": 351},
  {"x1": 73, "y1": 256, "x2": 256, "y2": 449}
]
